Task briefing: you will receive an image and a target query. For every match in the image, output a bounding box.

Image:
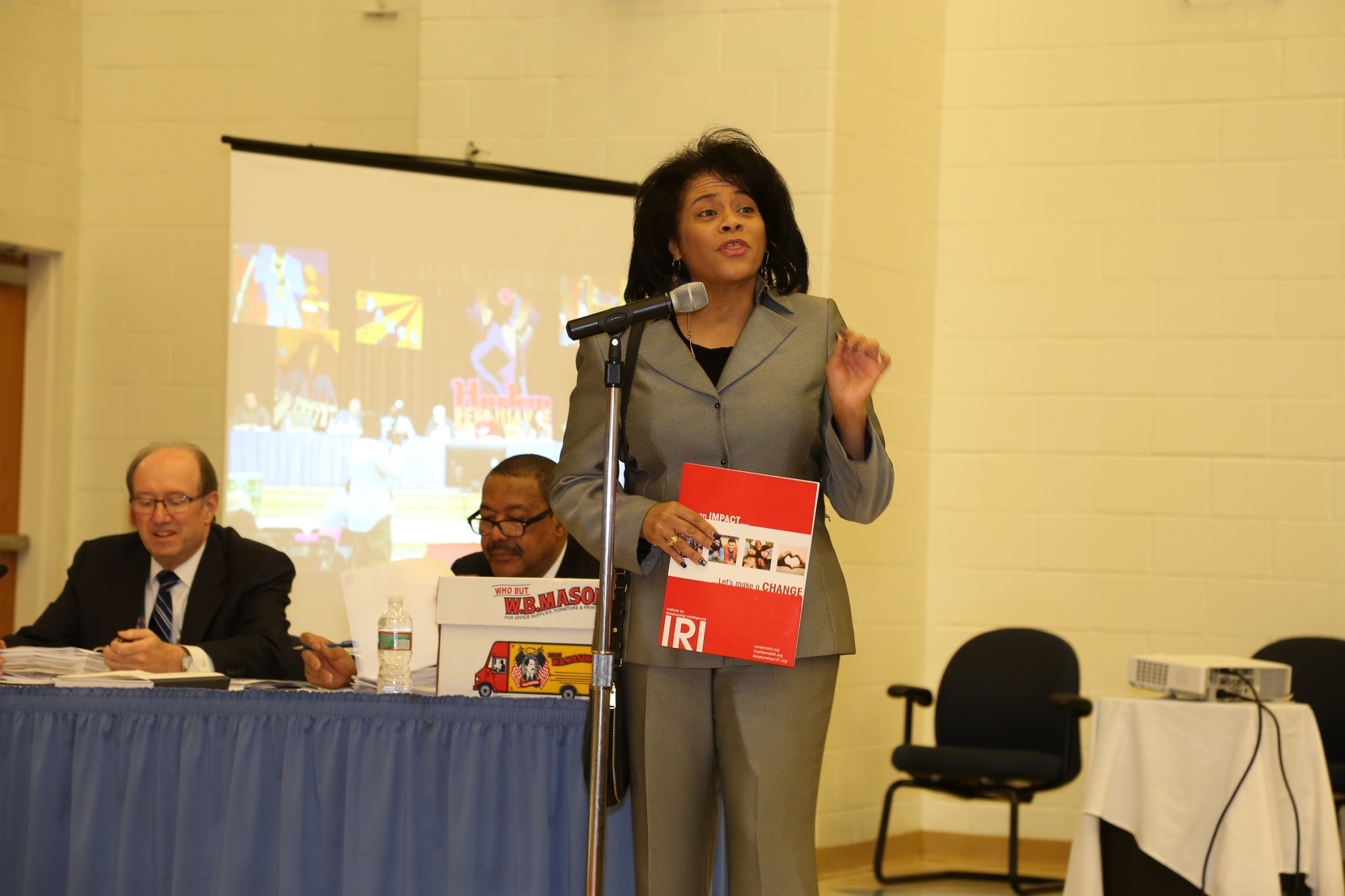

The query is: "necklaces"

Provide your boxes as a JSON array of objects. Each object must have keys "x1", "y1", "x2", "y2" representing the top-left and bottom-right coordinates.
[{"x1": 684, "y1": 311, "x2": 695, "y2": 357}]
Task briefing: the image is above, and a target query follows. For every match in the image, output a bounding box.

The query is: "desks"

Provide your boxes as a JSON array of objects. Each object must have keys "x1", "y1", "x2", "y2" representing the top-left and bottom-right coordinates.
[
  {"x1": 1062, "y1": 697, "x2": 1345, "y2": 896},
  {"x1": 0, "y1": 683, "x2": 727, "y2": 896}
]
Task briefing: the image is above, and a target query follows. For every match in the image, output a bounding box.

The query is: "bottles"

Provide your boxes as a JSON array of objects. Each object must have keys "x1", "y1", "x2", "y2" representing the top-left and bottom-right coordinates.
[{"x1": 377, "y1": 596, "x2": 413, "y2": 693}]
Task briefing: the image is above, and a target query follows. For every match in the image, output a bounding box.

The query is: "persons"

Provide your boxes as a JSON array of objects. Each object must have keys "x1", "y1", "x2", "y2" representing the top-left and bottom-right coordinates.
[
  {"x1": 546, "y1": 127, "x2": 896, "y2": 896},
  {"x1": 1, "y1": 443, "x2": 295, "y2": 685},
  {"x1": 675, "y1": 530, "x2": 806, "y2": 572},
  {"x1": 299, "y1": 455, "x2": 629, "y2": 687}
]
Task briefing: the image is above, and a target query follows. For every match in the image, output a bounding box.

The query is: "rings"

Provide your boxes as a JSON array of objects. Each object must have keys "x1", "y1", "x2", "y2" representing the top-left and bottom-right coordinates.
[{"x1": 666, "y1": 534, "x2": 679, "y2": 546}]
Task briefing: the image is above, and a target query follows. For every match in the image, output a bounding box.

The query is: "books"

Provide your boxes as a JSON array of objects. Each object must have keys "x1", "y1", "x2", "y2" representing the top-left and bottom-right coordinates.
[
  {"x1": 656, "y1": 462, "x2": 820, "y2": 669},
  {"x1": 55, "y1": 671, "x2": 229, "y2": 689}
]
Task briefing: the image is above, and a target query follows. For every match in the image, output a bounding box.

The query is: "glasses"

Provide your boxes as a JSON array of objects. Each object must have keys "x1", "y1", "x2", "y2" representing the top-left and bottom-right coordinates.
[
  {"x1": 129, "y1": 489, "x2": 214, "y2": 514},
  {"x1": 467, "y1": 507, "x2": 551, "y2": 537}
]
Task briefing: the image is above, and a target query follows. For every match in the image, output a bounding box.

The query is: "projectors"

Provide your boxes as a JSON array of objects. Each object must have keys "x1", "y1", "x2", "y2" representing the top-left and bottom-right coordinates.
[{"x1": 1130, "y1": 656, "x2": 1292, "y2": 701}]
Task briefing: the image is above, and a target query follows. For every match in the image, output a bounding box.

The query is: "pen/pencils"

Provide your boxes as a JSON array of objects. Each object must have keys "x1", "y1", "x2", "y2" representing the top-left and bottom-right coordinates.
[{"x1": 293, "y1": 641, "x2": 353, "y2": 650}]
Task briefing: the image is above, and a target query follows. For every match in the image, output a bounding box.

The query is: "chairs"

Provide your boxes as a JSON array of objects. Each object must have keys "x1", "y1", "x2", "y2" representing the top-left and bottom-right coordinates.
[
  {"x1": 1247, "y1": 638, "x2": 1345, "y2": 807},
  {"x1": 874, "y1": 628, "x2": 1092, "y2": 896}
]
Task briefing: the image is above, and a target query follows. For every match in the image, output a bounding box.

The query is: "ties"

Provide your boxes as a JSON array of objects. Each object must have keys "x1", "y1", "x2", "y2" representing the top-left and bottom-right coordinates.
[{"x1": 148, "y1": 570, "x2": 181, "y2": 643}]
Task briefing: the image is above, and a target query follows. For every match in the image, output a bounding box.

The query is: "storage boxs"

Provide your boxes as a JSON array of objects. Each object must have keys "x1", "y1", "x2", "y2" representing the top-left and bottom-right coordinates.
[{"x1": 435, "y1": 577, "x2": 600, "y2": 700}]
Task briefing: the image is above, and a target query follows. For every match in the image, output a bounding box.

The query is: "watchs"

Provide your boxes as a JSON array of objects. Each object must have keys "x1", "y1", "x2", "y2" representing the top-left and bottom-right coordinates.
[{"x1": 181, "y1": 645, "x2": 193, "y2": 671}]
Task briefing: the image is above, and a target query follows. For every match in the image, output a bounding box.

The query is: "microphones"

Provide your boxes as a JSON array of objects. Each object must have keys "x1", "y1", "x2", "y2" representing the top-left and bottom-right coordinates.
[{"x1": 566, "y1": 282, "x2": 709, "y2": 341}]
